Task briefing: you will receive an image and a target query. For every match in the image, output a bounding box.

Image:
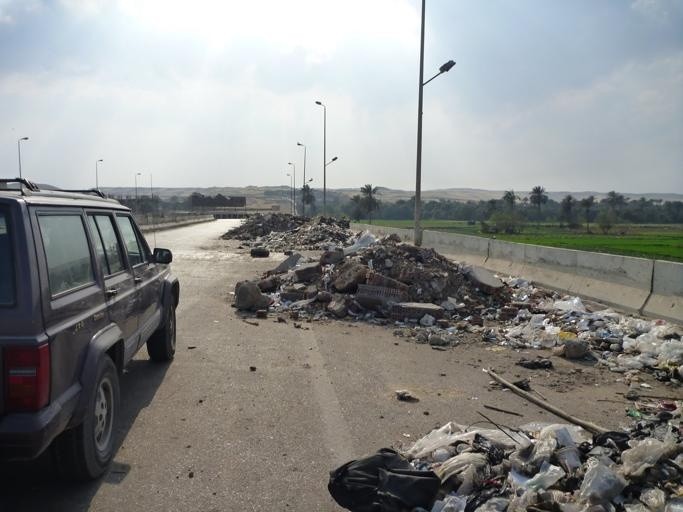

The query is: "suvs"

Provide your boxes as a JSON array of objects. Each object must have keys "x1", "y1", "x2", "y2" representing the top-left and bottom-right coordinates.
[{"x1": 0, "y1": 179, "x2": 180, "y2": 482}]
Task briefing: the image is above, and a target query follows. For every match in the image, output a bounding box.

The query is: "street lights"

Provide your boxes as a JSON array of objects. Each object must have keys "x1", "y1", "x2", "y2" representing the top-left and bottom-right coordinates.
[
  {"x1": 284, "y1": 161, "x2": 297, "y2": 218},
  {"x1": 96, "y1": 160, "x2": 105, "y2": 192},
  {"x1": 17, "y1": 137, "x2": 29, "y2": 181},
  {"x1": 134, "y1": 173, "x2": 141, "y2": 199},
  {"x1": 296, "y1": 143, "x2": 314, "y2": 219},
  {"x1": 414, "y1": 1, "x2": 457, "y2": 251},
  {"x1": 316, "y1": 101, "x2": 339, "y2": 218}
]
